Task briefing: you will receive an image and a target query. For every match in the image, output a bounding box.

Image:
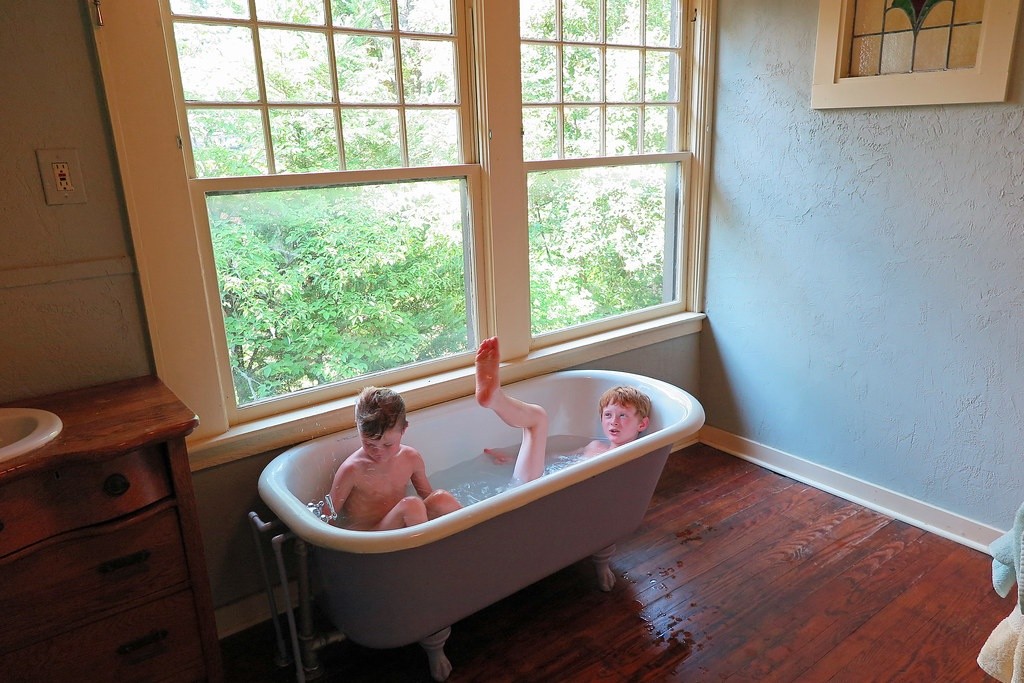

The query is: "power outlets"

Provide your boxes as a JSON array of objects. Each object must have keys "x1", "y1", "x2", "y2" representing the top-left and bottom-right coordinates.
[{"x1": 36, "y1": 147, "x2": 87, "y2": 206}]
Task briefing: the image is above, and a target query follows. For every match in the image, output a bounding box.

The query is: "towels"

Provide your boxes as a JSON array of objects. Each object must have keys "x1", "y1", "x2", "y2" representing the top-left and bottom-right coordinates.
[{"x1": 977, "y1": 502, "x2": 1024, "y2": 683}]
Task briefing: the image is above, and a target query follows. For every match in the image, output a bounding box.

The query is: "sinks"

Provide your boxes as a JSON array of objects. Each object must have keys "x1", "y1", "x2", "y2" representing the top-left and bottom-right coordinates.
[{"x1": 0, "y1": 408, "x2": 63, "y2": 464}]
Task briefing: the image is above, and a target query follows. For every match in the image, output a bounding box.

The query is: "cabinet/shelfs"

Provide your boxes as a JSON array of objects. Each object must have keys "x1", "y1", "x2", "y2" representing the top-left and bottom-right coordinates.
[{"x1": 0, "y1": 373, "x2": 226, "y2": 683}]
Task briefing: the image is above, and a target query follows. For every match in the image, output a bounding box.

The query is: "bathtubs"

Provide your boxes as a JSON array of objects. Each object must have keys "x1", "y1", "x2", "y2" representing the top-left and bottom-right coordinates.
[{"x1": 256, "y1": 369, "x2": 706, "y2": 651}]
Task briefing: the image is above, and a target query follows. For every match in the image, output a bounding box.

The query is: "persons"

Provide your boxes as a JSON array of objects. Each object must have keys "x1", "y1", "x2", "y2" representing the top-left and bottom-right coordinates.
[
  {"x1": 322, "y1": 386, "x2": 464, "y2": 531},
  {"x1": 475, "y1": 337, "x2": 651, "y2": 484}
]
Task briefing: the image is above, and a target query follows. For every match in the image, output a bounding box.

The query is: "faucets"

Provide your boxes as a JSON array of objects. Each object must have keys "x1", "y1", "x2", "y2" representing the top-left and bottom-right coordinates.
[{"x1": 306, "y1": 494, "x2": 337, "y2": 524}]
